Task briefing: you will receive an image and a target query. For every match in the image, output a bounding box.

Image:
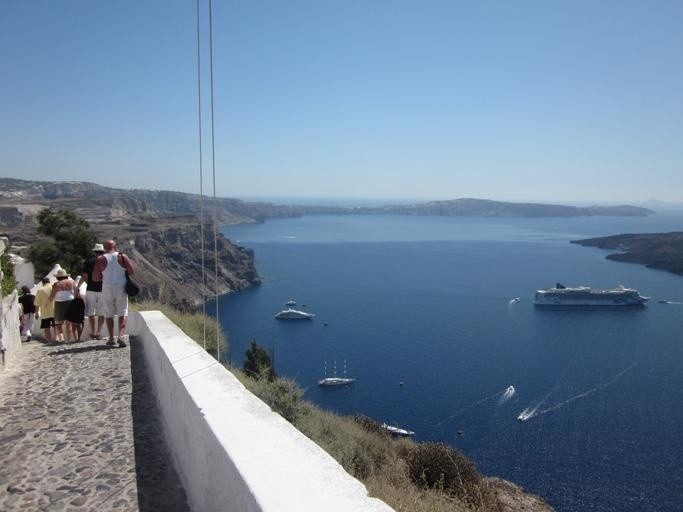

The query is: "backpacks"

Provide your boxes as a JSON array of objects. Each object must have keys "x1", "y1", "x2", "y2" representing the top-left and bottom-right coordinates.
[{"x1": 62, "y1": 294, "x2": 86, "y2": 331}]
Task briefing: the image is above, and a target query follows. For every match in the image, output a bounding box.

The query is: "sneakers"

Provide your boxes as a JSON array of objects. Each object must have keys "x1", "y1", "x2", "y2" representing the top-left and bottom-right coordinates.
[
  {"x1": 26, "y1": 333, "x2": 36, "y2": 342},
  {"x1": 90, "y1": 333, "x2": 103, "y2": 340},
  {"x1": 106, "y1": 339, "x2": 115, "y2": 348},
  {"x1": 44, "y1": 338, "x2": 68, "y2": 346},
  {"x1": 117, "y1": 336, "x2": 126, "y2": 348}
]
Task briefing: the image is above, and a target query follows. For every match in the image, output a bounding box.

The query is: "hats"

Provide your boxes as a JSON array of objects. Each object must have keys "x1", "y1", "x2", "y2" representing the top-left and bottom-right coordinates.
[
  {"x1": 91, "y1": 243, "x2": 107, "y2": 252},
  {"x1": 52, "y1": 270, "x2": 71, "y2": 277}
]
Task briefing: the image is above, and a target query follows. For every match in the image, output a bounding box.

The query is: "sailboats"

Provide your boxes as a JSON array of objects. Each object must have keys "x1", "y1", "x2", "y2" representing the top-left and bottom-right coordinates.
[{"x1": 317, "y1": 355, "x2": 355, "y2": 387}]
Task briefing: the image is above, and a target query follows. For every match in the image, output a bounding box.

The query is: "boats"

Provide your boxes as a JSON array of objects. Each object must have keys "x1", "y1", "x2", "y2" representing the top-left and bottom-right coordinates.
[
  {"x1": 517, "y1": 415, "x2": 526, "y2": 422},
  {"x1": 380, "y1": 422, "x2": 414, "y2": 435},
  {"x1": 656, "y1": 299, "x2": 667, "y2": 303},
  {"x1": 286, "y1": 300, "x2": 296, "y2": 305},
  {"x1": 273, "y1": 307, "x2": 316, "y2": 320},
  {"x1": 507, "y1": 385, "x2": 514, "y2": 393},
  {"x1": 510, "y1": 297, "x2": 521, "y2": 304}
]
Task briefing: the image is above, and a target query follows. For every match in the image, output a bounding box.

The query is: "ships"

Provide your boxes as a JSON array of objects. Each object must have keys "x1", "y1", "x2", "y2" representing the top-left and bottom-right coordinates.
[{"x1": 531, "y1": 282, "x2": 651, "y2": 306}]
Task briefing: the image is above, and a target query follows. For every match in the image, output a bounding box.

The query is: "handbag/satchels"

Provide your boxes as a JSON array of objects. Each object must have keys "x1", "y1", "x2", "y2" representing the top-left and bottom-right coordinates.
[{"x1": 118, "y1": 252, "x2": 141, "y2": 297}]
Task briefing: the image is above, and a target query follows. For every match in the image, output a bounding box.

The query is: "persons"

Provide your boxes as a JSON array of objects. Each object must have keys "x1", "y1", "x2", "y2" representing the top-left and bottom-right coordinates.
[
  {"x1": 92, "y1": 240, "x2": 135, "y2": 347},
  {"x1": 76, "y1": 243, "x2": 106, "y2": 340},
  {"x1": 18, "y1": 268, "x2": 81, "y2": 343}
]
